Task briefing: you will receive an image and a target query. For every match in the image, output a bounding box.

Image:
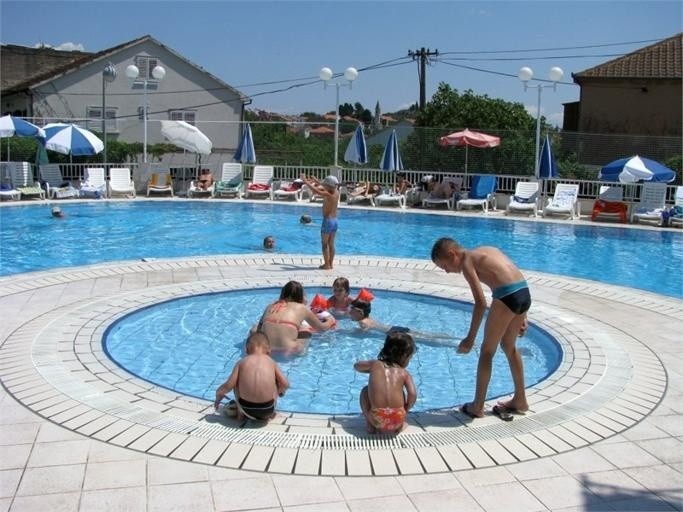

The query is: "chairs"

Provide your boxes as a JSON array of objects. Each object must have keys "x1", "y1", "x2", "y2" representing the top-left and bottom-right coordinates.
[
  {"x1": 630, "y1": 181, "x2": 667, "y2": 227},
  {"x1": 505, "y1": 182, "x2": 541, "y2": 218},
  {"x1": 188, "y1": 180, "x2": 214, "y2": 198},
  {"x1": 38, "y1": 164, "x2": 79, "y2": 199},
  {"x1": 307, "y1": 183, "x2": 325, "y2": 202},
  {"x1": 346, "y1": 193, "x2": 374, "y2": 208},
  {"x1": 592, "y1": 185, "x2": 627, "y2": 225},
  {"x1": 10, "y1": 162, "x2": 45, "y2": 200},
  {"x1": 215, "y1": 163, "x2": 242, "y2": 200},
  {"x1": 421, "y1": 177, "x2": 458, "y2": 210},
  {"x1": 375, "y1": 187, "x2": 419, "y2": 210},
  {"x1": 80, "y1": 168, "x2": 107, "y2": 200},
  {"x1": 245, "y1": 166, "x2": 274, "y2": 200},
  {"x1": 0, "y1": 163, "x2": 21, "y2": 201},
  {"x1": 541, "y1": 184, "x2": 581, "y2": 221},
  {"x1": 273, "y1": 184, "x2": 305, "y2": 202},
  {"x1": 668, "y1": 186, "x2": 683, "y2": 228},
  {"x1": 146, "y1": 163, "x2": 173, "y2": 197},
  {"x1": 456, "y1": 174, "x2": 497, "y2": 213},
  {"x1": 108, "y1": 168, "x2": 136, "y2": 200}
]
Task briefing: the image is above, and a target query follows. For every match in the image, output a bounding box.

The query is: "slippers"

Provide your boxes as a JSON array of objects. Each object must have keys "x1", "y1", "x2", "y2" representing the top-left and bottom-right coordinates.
[
  {"x1": 492, "y1": 406, "x2": 512, "y2": 421},
  {"x1": 462, "y1": 403, "x2": 483, "y2": 418}
]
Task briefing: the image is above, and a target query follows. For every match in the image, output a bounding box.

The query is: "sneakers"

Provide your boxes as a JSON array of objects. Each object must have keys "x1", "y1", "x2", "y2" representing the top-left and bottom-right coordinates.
[{"x1": 224, "y1": 400, "x2": 236, "y2": 417}]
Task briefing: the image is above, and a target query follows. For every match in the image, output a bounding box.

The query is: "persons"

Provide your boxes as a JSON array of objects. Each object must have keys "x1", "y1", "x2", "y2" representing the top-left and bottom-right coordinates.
[
  {"x1": 194, "y1": 168, "x2": 208, "y2": 192},
  {"x1": 205, "y1": 169, "x2": 214, "y2": 187},
  {"x1": 49, "y1": 207, "x2": 70, "y2": 219},
  {"x1": 299, "y1": 213, "x2": 323, "y2": 228},
  {"x1": 352, "y1": 330, "x2": 418, "y2": 435},
  {"x1": 277, "y1": 169, "x2": 463, "y2": 210},
  {"x1": 430, "y1": 237, "x2": 533, "y2": 419},
  {"x1": 213, "y1": 330, "x2": 289, "y2": 424},
  {"x1": 263, "y1": 236, "x2": 277, "y2": 252},
  {"x1": 339, "y1": 297, "x2": 475, "y2": 350},
  {"x1": 247, "y1": 279, "x2": 338, "y2": 360},
  {"x1": 298, "y1": 171, "x2": 341, "y2": 270}
]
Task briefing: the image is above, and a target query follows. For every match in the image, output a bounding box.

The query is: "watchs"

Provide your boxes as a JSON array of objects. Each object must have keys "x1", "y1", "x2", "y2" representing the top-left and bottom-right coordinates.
[{"x1": 323, "y1": 276, "x2": 358, "y2": 318}]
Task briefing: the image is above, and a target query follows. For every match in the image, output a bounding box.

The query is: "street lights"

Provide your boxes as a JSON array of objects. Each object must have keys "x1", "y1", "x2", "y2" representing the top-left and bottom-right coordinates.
[
  {"x1": 126, "y1": 63, "x2": 166, "y2": 163},
  {"x1": 519, "y1": 65, "x2": 565, "y2": 179},
  {"x1": 319, "y1": 66, "x2": 358, "y2": 169}
]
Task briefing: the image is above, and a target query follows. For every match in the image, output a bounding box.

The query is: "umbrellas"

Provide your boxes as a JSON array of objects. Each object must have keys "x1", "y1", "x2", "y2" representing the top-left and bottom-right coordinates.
[
  {"x1": 229, "y1": 121, "x2": 258, "y2": 192},
  {"x1": 343, "y1": 121, "x2": 368, "y2": 198},
  {"x1": 0, "y1": 112, "x2": 47, "y2": 161},
  {"x1": 378, "y1": 126, "x2": 404, "y2": 197},
  {"x1": 433, "y1": 124, "x2": 502, "y2": 193},
  {"x1": 532, "y1": 131, "x2": 560, "y2": 210},
  {"x1": 36, "y1": 119, "x2": 106, "y2": 189},
  {"x1": 153, "y1": 116, "x2": 214, "y2": 190},
  {"x1": 595, "y1": 152, "x2": 676, "y2": 223}
]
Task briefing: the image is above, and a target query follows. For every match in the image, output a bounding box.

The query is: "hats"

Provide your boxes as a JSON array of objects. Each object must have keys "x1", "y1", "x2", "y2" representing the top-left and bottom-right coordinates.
[
  {"x1": 322, "y1": 175, "x2": 339, "y2": 188},
  {"x1": 350, "y1": 300, "x2": 370, "y2": 316}
]
